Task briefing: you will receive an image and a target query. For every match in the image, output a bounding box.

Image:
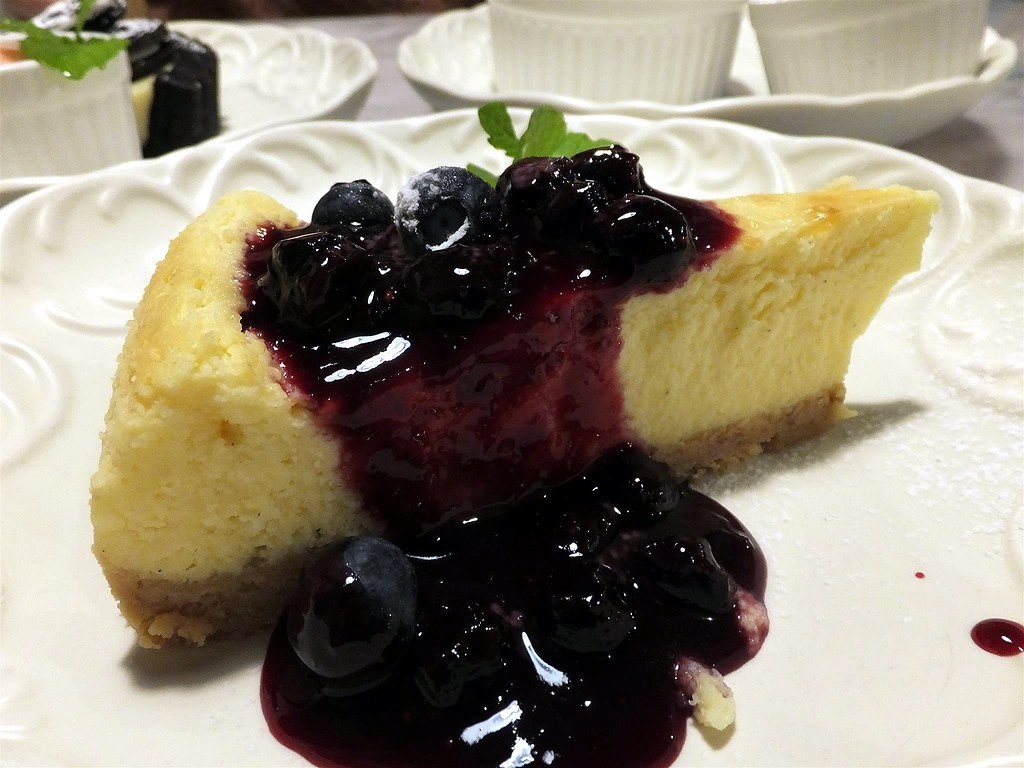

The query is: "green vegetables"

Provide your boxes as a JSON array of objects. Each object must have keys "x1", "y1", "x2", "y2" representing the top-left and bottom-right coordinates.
[{"x1": 1, "y1": 1, "x2": 132, "y2": 79}]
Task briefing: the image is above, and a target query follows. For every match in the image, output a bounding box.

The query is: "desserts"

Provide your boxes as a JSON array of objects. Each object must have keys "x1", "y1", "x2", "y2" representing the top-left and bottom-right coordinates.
[
  {"x1": 32, "y1": 0, "x2": 221, "y2": 157},
  {"x1": 91, "y1": 146, "x2": 932, "y2": 651}
]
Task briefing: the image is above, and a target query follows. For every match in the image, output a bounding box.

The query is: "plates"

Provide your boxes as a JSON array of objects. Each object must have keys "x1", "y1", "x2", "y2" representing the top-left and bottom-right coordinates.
[
  {"x1": 0, "y1": 17, "x2": 377, "y2": 196},
  {"x1": 0, "y1": 100, "x2": 1024, "y2": 768},
  {"x1": 396, "y1": 4, "x2": 1017, "y2": 147}
]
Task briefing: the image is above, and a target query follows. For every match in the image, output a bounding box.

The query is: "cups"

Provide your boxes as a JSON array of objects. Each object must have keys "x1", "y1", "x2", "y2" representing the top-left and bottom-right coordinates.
[
  {"x1": 747, "y1": 0, "x2": 990, "y2": 93},
  {"x1": 0, "y1": 33, "x2": 144, "y2": 179},
  {"x1": 488, "y1": 0, "x2": 742, "y2": 98}
]
